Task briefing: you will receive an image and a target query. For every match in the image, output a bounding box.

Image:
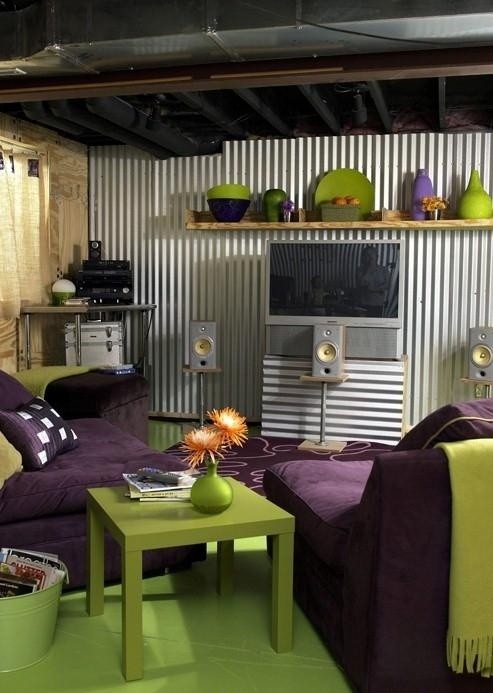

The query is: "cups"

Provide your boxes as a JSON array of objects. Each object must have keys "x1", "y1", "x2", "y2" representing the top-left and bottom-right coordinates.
[{"x1": 280, "y1": 208, "x2": 292, "y2": 222}]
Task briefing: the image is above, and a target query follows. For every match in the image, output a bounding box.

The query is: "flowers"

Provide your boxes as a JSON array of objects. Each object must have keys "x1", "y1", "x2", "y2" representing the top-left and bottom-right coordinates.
[
  {"x1": 179, "y1": 405, "x2": 250, "y2": 470},
  {"x1": 421, "y1": 194, "x2": 450, "y2": 212},
  {"x1": 280, "y1": 199, "x2": 294, "y2": 214}
]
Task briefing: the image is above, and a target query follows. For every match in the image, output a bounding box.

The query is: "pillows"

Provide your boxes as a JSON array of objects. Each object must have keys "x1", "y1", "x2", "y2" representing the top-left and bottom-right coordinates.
[{"x1": 0, "y1": 394, "x2": 82, "y2": 470}]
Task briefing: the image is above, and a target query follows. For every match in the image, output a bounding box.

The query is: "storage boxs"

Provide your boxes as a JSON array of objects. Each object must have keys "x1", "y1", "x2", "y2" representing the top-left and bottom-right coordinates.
[{"x1": 63, "y1": 320, "x2": 124, "y2": 366}]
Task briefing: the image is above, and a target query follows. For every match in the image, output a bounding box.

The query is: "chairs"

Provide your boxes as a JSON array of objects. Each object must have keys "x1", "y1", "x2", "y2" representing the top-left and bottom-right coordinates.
[
  {"x1": 260, "y1": 395, "x2": 493, "y2": 691},
  {"x1": 0, "y1": 366, "x2": 207, "y2": 595}
]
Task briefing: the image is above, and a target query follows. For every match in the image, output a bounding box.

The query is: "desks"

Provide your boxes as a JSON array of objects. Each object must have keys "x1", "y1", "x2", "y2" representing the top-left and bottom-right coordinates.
[
  {"x1": 295, "y1": 372, "x2": 349, "y2": 453},
  {"x1": 82, "y1": 475, "x2": 299, "y2": 683},
  {"x1": 182, "y1": 363, "x2": 222, "y2": 437},
  {"x1": 86, "y1": 303, "x2": 158, "y2": 375},
  {"x1": 21, "y1": 304, "x2": 89, "y2": 368},
  {"x1": 458, "y1": 377, "x2": 492, "y2": 400}
]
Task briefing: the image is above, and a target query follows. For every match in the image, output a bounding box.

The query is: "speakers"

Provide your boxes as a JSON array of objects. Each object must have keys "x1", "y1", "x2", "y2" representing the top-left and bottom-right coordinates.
[
  {"x1": 468, "y1": 327, "x2": 493, "y2": 381},
  {"x1": 88, "y1": 241, "x2": 102, "y2": 260},
  {"x1": 312, "y1": 324, "x2": 344, "y2": 376},
  {"x1": 189, "y1": 320, "x2": 217, "y2": 369}
]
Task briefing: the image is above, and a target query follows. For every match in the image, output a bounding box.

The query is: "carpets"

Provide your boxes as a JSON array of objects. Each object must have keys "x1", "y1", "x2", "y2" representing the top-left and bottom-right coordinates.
[{"x1": 163, "y1": 435, "x2": 395, "y2": 498}]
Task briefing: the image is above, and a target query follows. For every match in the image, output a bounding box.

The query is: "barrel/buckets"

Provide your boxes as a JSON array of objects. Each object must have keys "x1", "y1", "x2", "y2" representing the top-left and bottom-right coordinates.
[{"x1": 0, "y1": 557, "x2": 67, "y2": 673}]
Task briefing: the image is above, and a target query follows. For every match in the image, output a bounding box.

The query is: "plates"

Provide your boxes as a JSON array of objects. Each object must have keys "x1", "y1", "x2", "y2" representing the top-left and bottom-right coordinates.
[{"x1": 313, "y1": 168, "x2": 375, "y2": 216}]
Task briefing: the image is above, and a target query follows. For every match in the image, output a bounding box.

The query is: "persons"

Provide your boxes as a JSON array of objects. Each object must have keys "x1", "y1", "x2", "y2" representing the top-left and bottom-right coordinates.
[
  {"x1": 308, "y1": 275, "x2": 331, "y2": 306},
  {"x1": 353, "y1": 247, "x2": 392, "y2": 317}
]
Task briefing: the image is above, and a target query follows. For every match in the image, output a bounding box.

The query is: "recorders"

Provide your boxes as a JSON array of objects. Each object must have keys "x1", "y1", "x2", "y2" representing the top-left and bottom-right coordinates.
[{"x1": 73, "y1": 269, "x2": 134, "y2": 304}]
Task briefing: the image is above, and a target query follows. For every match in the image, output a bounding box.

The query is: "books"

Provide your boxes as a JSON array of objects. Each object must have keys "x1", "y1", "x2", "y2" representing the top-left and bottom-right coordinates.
[
  {"x1": 122, "y1": 471, "x2": 198, "y2": 501},
  {"x1": 0, "y1": 547, "x2": 65, "y2": 598}
]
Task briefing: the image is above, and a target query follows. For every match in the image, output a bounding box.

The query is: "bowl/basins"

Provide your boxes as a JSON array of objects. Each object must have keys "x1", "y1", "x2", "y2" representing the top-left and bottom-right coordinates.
[{"x1": 207, "y1": 183, "x2": 251, "y2": 222}]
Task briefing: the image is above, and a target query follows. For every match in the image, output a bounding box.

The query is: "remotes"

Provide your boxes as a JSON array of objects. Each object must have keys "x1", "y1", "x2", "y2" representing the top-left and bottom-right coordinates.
[{"x1": 138, "y1": 467, "x2": 184, "y2": 484}]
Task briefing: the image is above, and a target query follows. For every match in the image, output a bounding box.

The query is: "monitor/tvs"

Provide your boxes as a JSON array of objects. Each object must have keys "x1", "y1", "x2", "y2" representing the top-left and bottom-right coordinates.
[{"x1": 265, "y1": 240, "x2": 405, "y2": 328}]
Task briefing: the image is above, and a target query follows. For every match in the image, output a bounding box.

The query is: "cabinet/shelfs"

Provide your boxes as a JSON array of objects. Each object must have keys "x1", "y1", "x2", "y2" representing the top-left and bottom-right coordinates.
[{"x1": 259, "y1": 352, "x2": 409, "y2": 445}]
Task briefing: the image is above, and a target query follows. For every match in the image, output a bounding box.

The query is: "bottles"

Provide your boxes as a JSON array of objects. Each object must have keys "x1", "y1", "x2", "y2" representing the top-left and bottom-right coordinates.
[{"x1": 456, "y1": 170, "x2": 493, "y2": 218}]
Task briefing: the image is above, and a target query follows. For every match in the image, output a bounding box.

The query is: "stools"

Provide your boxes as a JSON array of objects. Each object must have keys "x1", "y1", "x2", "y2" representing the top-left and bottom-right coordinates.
[{"x1": 9, "y1": 363, "x2": 150, "y2": 448}]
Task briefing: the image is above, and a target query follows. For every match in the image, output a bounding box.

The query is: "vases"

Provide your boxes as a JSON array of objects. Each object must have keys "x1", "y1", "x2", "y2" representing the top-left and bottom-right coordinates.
[
  {"x1": 426, "y1": 209, "x2": 441, "y2": 221},
  {"x1": 263, "y1": 187, "x2": 287, "y2": 221},
  {"x1": 282, "y1": 208, "x2": 292, "y2": 222},
  {"x1": 189, "y1": 457, "x2": 233, "y2": 514},
  {"x1": 454, "y1": 167, "x2": 491, "y2": 221}
]
